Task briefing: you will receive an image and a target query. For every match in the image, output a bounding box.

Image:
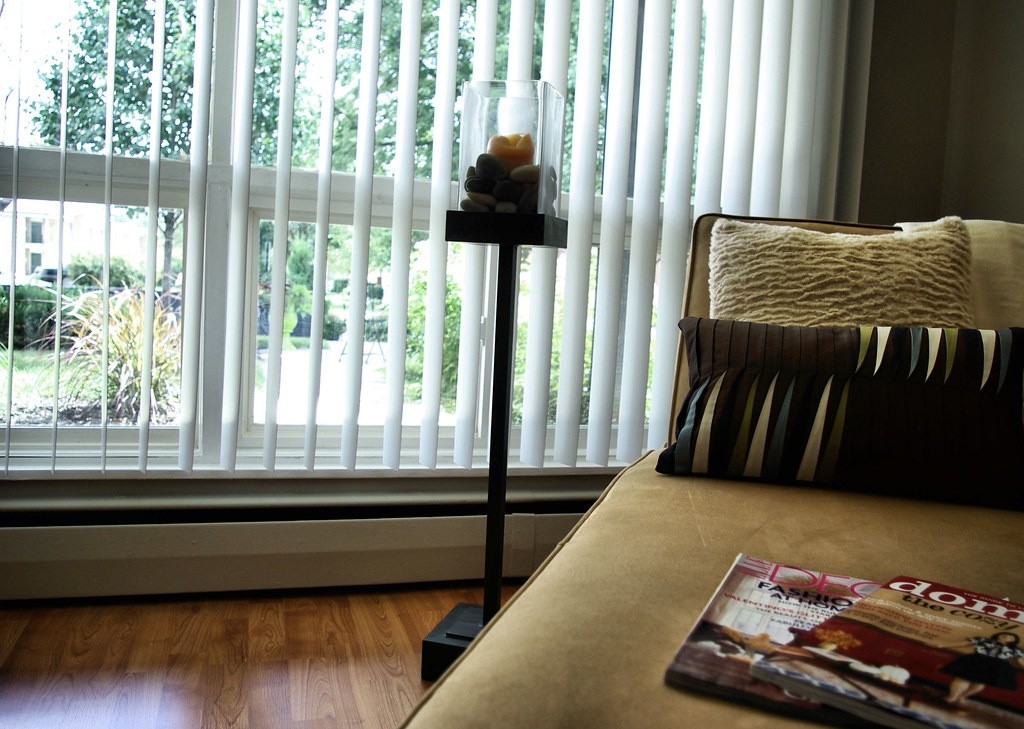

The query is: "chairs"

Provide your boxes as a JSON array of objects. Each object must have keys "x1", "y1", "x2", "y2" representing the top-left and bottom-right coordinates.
[{"x1": 399, "y1": 214, "x2": 1024, "y2": 729}]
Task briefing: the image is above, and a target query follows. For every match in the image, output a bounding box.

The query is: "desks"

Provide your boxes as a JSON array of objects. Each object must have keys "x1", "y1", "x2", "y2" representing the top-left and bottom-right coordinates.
[{"x1": 337, "y1": 317, "x2": 388, "y2": 366}]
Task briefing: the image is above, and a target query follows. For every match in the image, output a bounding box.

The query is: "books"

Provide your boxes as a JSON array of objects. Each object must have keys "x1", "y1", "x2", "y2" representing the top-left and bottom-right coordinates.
[{"x1": 665, "y1": 553, "x2": 1024, "y2": 729}]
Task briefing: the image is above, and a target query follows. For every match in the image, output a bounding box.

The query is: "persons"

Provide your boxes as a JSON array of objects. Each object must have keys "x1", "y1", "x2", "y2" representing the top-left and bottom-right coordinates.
[{"x1": 937, "y1": 632, "x2": 1024, "y2": 716}]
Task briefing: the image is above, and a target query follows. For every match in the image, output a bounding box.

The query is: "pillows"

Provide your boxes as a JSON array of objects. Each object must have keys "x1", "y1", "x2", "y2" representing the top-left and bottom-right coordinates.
[
  {"x1": 708, "y1": 215, "x2": 977, "y2": 328},
  {"x1": 654, "y1": 316, "x2": 1024, "y2": 512}
]
